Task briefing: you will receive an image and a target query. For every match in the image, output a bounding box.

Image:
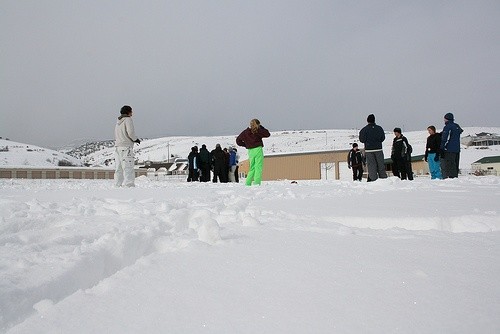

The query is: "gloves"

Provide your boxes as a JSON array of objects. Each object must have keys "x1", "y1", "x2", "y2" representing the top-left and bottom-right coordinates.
[
  {"x1": 434, "y1": 155, "x2": 440, "y2": 161},
  {"x1": 136, "y1": 139, "x2": 141, "y2": 144}
]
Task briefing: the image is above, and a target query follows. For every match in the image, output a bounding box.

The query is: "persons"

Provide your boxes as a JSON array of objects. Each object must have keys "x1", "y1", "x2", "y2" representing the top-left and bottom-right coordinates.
[
  {"x1": 236, "y1": 119, "x2": 271, "y2": 185},
  {"x1": 114, "y1": 105, "x2": 140, "y2": 188},
  {"x1": 359, "y1": 114, "x2": 388, "y2": 181},
  {"x1": 187, "y1": 144, "x2": 240, "y2": 183},
  {"x1": 425, "y1": 126, "x2": 443, "y2": 178},
  {"x1": 440, "y1": 112, "x2": 464, "y2": 178},
  {"x1": 347, "y1": 143, "x2": 366, "y2": 181},
  {"x1": 391, "y1": 127, "x2": 414, "y2": 180}
]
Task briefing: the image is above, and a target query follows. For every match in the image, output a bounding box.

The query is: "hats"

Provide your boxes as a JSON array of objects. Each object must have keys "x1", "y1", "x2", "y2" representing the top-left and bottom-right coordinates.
[
  {"x1": 367, "y1": 114, "x2": 375, "y2": 123},
  {"x1": 444, "y1": 113, "x2": 454, "y2": 121}
]
